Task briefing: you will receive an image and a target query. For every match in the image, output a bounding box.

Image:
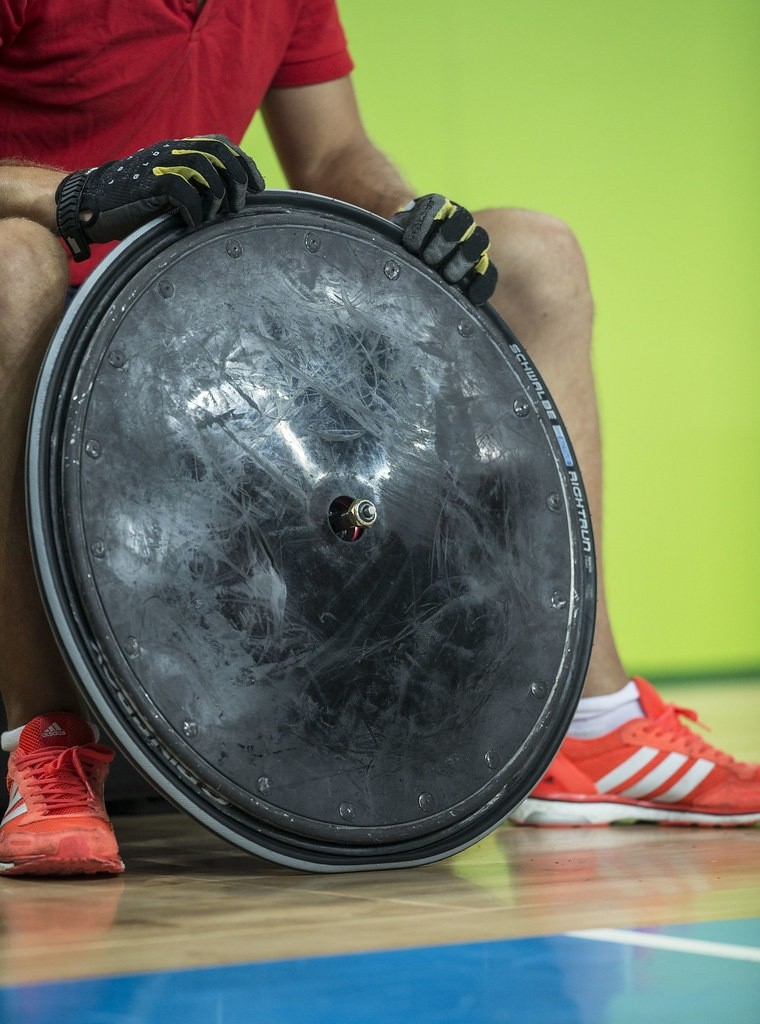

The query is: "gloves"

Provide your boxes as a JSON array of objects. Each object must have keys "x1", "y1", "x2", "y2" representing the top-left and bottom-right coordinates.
[
  {"x1": 54, "y1": 133, "x2": 263, "y2": 264},
  {"x1": 389, "y1": 193, "x2": 498, "y2": 306}
]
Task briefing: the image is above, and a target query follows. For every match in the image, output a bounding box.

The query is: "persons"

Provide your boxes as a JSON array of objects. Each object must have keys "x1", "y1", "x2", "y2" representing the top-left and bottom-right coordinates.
[{"x1": 0, "y1": 0, "x2": 760, "y2": 876}]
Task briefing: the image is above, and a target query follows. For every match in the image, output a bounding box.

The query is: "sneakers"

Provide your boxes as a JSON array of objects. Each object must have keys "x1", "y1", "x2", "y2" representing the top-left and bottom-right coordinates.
[
  {"x1": 0, "y1": 714, "x2": 126, "y2": 875},
  {"x1": 507, "y1": 674, "x2": 760, "y2": 827}
]
